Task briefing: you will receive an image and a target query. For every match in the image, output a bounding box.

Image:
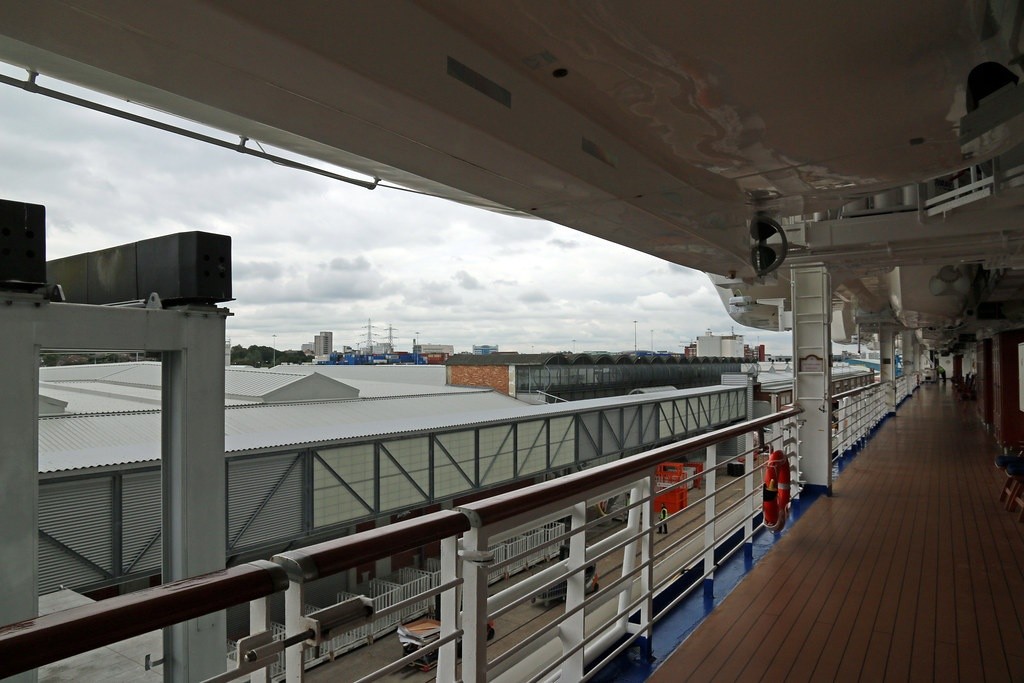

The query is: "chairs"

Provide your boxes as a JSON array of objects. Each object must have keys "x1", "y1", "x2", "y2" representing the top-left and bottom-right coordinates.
[{"x1": 994, "y1": 450, "x2": 1024, "y2": 523}]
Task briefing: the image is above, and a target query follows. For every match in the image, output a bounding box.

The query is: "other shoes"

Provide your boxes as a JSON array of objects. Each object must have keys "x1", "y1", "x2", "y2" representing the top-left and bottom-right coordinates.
[
  {"x1": 663, "y1": 532, "x2": 667, "y2": 534},
  {"x1": 657, "y1": 532, "x2": 662, "y2": 534},
  {"x1": 942, "y1": 380, "x2": 946, "y2": 382}
]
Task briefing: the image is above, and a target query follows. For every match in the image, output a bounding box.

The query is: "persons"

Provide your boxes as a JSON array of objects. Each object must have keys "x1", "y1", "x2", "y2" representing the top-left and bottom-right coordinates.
[
  {"x1": 657, "y1": 503, "x2": 669, "y2": 534},
  {"x1": 625, "y1": 494, "x2": 630, "y2": 506},
  {"x1": 936, "y1": 365, "x2": 947, "y2": 383}
]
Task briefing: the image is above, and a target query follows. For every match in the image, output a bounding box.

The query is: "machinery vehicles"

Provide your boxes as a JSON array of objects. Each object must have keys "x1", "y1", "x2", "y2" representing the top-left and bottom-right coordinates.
[
  {"x1": 531, "y1": 544, "x2": 598, "y2": 608},
  {"x1": 397, "y1": 605, "x2": 495, "y2": 672}
]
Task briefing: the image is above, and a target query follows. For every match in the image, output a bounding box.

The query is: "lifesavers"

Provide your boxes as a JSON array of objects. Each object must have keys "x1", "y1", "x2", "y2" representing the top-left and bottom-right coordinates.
[{"x1": 763, "y1": 449, "x2": 791, "y2": 532}]
